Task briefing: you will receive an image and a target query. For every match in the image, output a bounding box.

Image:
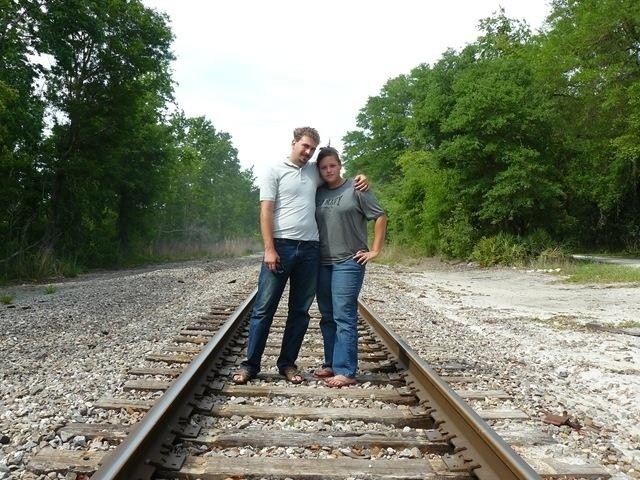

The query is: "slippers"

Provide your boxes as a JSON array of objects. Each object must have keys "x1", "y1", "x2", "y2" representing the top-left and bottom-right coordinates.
[
  {"x1": 313, "y1": 365, "x2": 336, "y2": 378},
  {"x1": 323, "y1": 372, "x2": 358, "y2": 388}
]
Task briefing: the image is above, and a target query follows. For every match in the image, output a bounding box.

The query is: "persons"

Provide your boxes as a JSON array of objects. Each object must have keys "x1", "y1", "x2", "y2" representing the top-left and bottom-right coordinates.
[
  {"x1": 230, "y1": 125, "x2": 372, "y2": 385},
  {"x1": 314, "y1": 145, "x2": 388, "y2": 387}
]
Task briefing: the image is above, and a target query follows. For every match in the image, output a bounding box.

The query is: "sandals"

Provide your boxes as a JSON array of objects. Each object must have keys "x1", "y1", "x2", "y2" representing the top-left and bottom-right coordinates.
[
  {"x1": 231, "y1": 366, "x2": 259, "y2": 385},
  {"x1": 278, "y1": 365, "x2": 305, "y2": 384}
]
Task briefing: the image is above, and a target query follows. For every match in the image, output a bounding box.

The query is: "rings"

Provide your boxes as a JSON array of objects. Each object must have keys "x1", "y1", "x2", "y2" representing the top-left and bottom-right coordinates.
[
  {"x1": 365, "y1": 255, "x2": 367, "y2": 258},
  {"x1": 365, "y1": 183, "x2": 369, "y2": 188}
]
